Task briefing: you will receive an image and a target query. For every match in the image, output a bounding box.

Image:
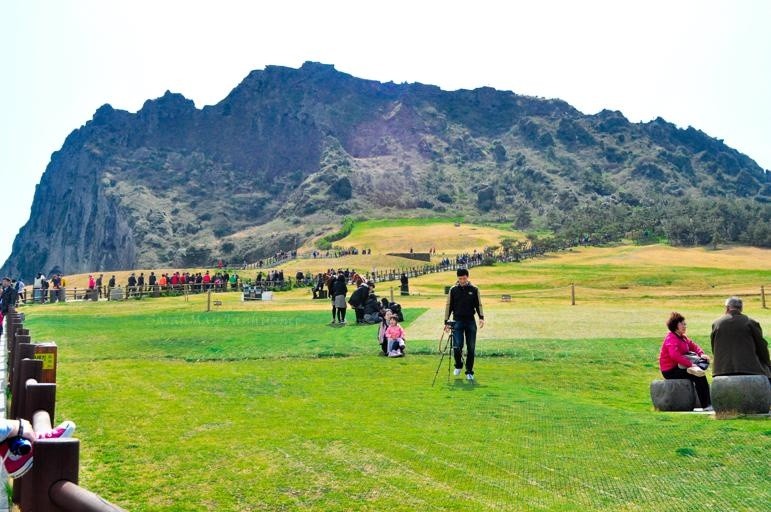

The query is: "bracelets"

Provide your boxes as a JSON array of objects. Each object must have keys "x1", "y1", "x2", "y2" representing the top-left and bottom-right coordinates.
[{"x1": 17, "y1": 417, "x2": 24, "y2": 436}]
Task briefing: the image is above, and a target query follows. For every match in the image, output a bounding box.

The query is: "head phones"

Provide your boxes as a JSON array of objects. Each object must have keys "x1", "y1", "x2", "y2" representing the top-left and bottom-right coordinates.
[{"x1": 675, "y1": 335, "x2": 687, "y2": 343}]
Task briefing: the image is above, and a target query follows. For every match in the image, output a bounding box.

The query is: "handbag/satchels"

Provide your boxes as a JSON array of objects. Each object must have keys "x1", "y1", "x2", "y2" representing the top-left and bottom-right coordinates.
[{"x1": 678, "y1": 353, "x2": 708, "y2": 369}]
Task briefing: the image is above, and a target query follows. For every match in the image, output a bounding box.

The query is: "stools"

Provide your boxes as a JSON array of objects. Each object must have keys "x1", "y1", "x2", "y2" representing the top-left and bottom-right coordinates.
[
  {"x1": 649, "y1": 379, "x2": 696, "y2": 411},
  {"x1": 709, "y1": 375, "x2": 771, "y2": 415}
]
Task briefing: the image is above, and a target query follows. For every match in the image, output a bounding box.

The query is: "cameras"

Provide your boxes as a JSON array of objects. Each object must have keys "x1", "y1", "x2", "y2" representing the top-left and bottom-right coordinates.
[
  {"x1": 445, "y1": 320, "x2": 456, "y2": 326},
  {"x1": 8, "y1": 436, "x2": 32, "y2": 455}
]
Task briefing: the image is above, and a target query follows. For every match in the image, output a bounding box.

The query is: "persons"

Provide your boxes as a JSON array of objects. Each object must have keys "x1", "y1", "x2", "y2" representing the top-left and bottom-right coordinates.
[
  {"x1": 244, "y1": 259, "x2": 248, "y2": 269},
  {"x1": 313, "y1": 267, "x2": 405, "y2": 324},
  {"x1": 443, "y1": 267, "x2": 485, "y2": 380},
  {"x1": 306, "y1": 269, "x2": 313, "y2": 284},
  {"x1": 441, "y1": 249, "x2": 517, "y2": 267},
  {"x1": 710, "y1": 296, "x2": 771, "y2": 384},
  {"x1": 658, "y1": 311, "x2": 714, "y2": 411},
  {"x1": 385, "y1": 316, "x2": 406, "y2": 354},
  {"x1": 269, "y1": 246, "x2": 372, "y2": 259},
  {"x1": 84, "y1": 269, "x2": 285, "y2": 299},
  {"x1": 378, "y1": 309, "x2": 406, "y2": 355},
  {"x1": 218, "y1": 259, "x2": 223, "y2": 268},
  {"x1": 0, "y1": 271, "x2": 65, "y2": 334},
  {"x1": 259, "y1": 258, "x2": 263, "y2": 267}
]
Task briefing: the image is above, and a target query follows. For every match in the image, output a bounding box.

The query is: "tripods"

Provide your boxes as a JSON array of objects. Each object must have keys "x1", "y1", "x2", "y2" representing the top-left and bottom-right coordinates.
[{"x1": 431, "y1": 335, "x2": 473, "y2": 385}]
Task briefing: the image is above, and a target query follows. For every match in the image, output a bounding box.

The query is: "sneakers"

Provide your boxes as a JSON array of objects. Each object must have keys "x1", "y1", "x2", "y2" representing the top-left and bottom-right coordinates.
[
  {"x1": 466, "y1": 374, "x2": 474, "y2": 380},
  {"x1": 454, "y1": 368, "x2": 461, "y2": 376},
  {"x1": 703, "y1": 405, "x2": 714, "y2": 411},
  {"x1": 687, "y1": 366, "x2": 706, "y2": 378}
]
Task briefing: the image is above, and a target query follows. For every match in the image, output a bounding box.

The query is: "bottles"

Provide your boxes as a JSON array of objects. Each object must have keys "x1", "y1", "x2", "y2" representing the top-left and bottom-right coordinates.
[{"x1": 7, "y1": 436, "x2": 33, "y2": 456}]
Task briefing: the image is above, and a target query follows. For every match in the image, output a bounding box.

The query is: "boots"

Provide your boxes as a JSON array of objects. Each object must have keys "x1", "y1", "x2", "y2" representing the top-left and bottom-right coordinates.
[{"x1": 390, "y1": 349, "x2": 403, "y2": 357}]
